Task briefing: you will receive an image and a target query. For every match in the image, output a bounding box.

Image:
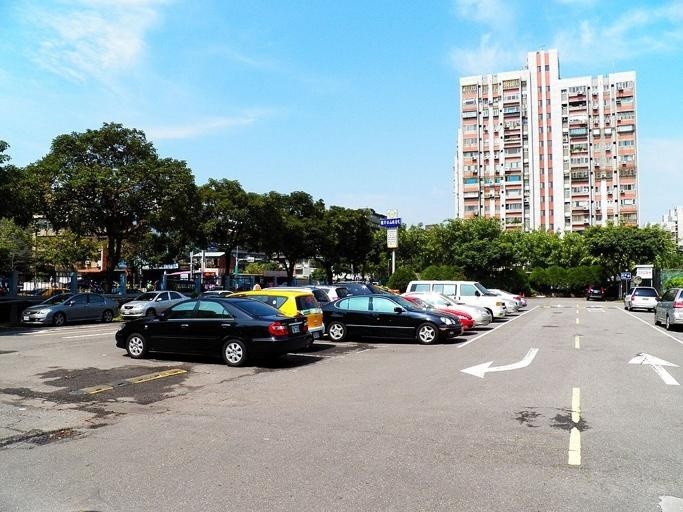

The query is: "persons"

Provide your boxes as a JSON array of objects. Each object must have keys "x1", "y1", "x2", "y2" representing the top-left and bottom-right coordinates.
[
  {"x1": 88, "y1": 279, "x2": 105, "y2": 292},
  {"x1": 144, "y1": 280, "x2": 160, "y2": 291},
  {"x1": 203, "y1": 281, "x2": 215, "y2": 290}
]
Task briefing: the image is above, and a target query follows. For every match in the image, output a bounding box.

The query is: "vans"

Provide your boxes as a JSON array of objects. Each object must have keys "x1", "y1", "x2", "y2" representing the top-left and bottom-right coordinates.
[{"x1": 652, "y1": 287, "x2": 682, "y2": 331}]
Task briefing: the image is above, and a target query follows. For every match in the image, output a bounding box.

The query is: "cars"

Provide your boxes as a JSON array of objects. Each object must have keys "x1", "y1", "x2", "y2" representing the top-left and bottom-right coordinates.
[
  {"x1": 622, "y1": 286, "x2": 662, "y2": 314},
  {"x1": 18, "y1": 277, "x2": 231, "y2": 326},
  {"x1": 113, "y1": 296, "x2": 311, "y2": 366}
]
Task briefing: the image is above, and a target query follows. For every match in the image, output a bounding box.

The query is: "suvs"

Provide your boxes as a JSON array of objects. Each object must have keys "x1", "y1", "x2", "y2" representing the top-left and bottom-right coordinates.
[{"x1": 585, "y1": 284, "x2": 606, "y2": 302}]
